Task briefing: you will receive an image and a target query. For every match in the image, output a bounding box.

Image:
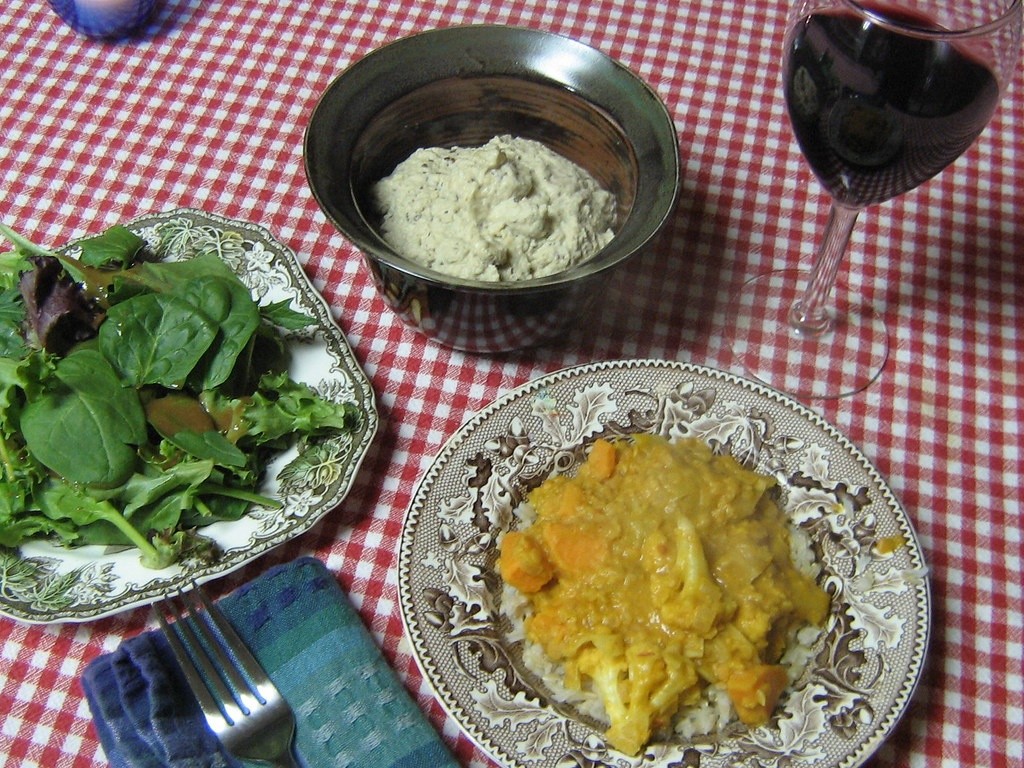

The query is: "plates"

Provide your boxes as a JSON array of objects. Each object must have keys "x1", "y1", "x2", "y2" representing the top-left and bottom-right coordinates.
[
  {"x1": 0, "y1": 209, "x2": 378, "y2": 625},
  {"x1": 399, "y1": 362, "x2": 929, "y2": 768}
]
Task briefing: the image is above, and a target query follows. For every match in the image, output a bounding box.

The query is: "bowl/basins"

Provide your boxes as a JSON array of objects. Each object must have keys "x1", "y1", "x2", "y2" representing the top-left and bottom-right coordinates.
[{"x1": 303, "y1": 27, "x2": 681, "y2": 352}]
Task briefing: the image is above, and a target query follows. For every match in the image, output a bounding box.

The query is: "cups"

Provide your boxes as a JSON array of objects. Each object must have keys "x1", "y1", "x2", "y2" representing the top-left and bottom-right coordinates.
[{"x1": 47, "y1": 0, "x2": 158, "y2": 40}]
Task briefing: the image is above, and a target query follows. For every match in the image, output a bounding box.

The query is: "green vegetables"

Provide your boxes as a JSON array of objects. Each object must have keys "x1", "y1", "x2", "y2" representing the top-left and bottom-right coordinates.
[{"x1": 0, "y1": 222, "x2": 344, "y2": 570}]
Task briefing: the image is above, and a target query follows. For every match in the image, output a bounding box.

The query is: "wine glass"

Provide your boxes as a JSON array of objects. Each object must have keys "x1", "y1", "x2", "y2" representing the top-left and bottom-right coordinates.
[{"x1": 725, "y1": 0, "x2": 1024, "y2": 396}]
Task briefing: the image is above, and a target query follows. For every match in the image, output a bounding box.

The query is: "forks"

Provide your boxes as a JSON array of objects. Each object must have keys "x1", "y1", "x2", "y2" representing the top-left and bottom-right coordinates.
[{"x1": 152, "y1": 579, "x2": 298, "y2": 768}]
